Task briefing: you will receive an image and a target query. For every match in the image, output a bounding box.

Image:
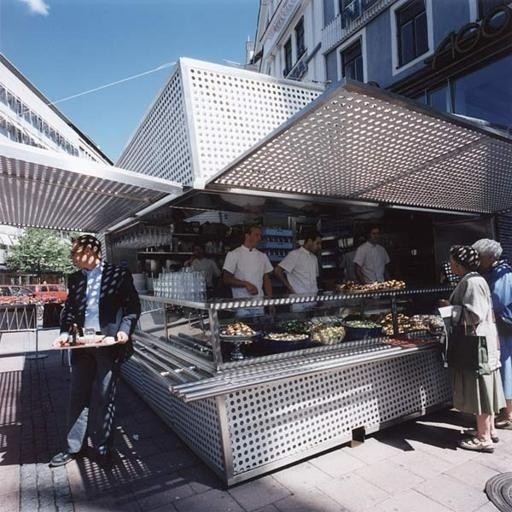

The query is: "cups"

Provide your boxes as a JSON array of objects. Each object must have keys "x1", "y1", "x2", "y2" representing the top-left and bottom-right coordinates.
[
  {"x1": 152, "y1": 266, "x2": 208, "y2": 302},
  {"x1": 83, "y1": 327, "x2": 95, "y2": 345}
]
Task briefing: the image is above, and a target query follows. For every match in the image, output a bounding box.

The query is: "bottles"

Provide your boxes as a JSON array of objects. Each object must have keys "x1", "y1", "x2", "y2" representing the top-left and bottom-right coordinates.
[{"x1": 68, "y1": 319, "x2": 77, "y2": 347}]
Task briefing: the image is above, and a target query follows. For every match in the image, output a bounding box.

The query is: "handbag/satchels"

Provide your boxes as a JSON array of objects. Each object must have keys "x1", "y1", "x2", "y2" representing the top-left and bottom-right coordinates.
[{"x1": 445, "y1": 305, "x2": 491, "y2": 377}]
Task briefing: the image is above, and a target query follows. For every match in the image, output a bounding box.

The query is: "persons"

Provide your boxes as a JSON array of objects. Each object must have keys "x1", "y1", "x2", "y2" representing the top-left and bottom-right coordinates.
[
  {"x1": 469, "y1": 237, "x2": 512, "y2": 432},
  {"x1": 337, "y1": 234, "x2": 368, "y2": 285},
  {"x1": 273, "y1": 229, "x2": 342, "y2": 314},
  {"x1": 353, "y1": 224, "x2": 393, "y2": 283},
  {"x1": 436, "y1": 244, "x2": 508, "y2": 454},
  {"x1": 47, "y1": 234, "x2": 144, "y2": 473},
  {"x1": 183, "y1": 241, "x2": 222, "y2": 298},
  {"x1": 222, "y1": 222, "x2": 277, "y2": 320}
]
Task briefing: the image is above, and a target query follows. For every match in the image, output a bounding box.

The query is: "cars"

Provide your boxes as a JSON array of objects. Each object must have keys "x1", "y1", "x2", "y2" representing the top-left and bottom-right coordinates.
[{"x1": 0, "y1": 280, "x2": 69, "y2": 302}]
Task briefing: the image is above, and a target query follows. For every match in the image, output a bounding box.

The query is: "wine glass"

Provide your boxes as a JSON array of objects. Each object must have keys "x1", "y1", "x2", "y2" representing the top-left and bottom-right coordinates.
[{"x1": 115, "y1": 225, "x2": 173, "y2": 250}]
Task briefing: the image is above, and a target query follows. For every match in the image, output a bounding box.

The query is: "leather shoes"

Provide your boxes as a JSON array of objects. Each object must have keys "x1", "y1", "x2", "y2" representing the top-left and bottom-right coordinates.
[
  {"x1": 49, "y1": 449, "x2": 80, "y2": 467},
  {"x1": 96, "y1": 444, "x2": 112, "y2": 468}
]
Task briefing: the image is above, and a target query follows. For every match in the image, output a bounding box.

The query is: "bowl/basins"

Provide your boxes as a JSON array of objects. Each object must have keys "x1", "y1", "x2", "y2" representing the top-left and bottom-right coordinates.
[
  {"x1": 262, "y1": 329, "x2": 311, "y2": 354},
  {"x1": 131, "y1": 271, "x2": 148, "y2": 295},
  {"x1": 344, "y1": 320, "x2": 383, "y2": 339}
]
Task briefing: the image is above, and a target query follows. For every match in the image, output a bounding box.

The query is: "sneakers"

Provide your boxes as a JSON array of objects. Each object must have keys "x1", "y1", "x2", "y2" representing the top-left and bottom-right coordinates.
[
  {"x1": 463, "y1": 429, "x2": 499, "y2": 443},
  {"x1": 456, "y1": 437, "x2": 494, "y2": 453},
  {"x1": 494, "y1": 415, "x2": 512, "y2": 429}
]
